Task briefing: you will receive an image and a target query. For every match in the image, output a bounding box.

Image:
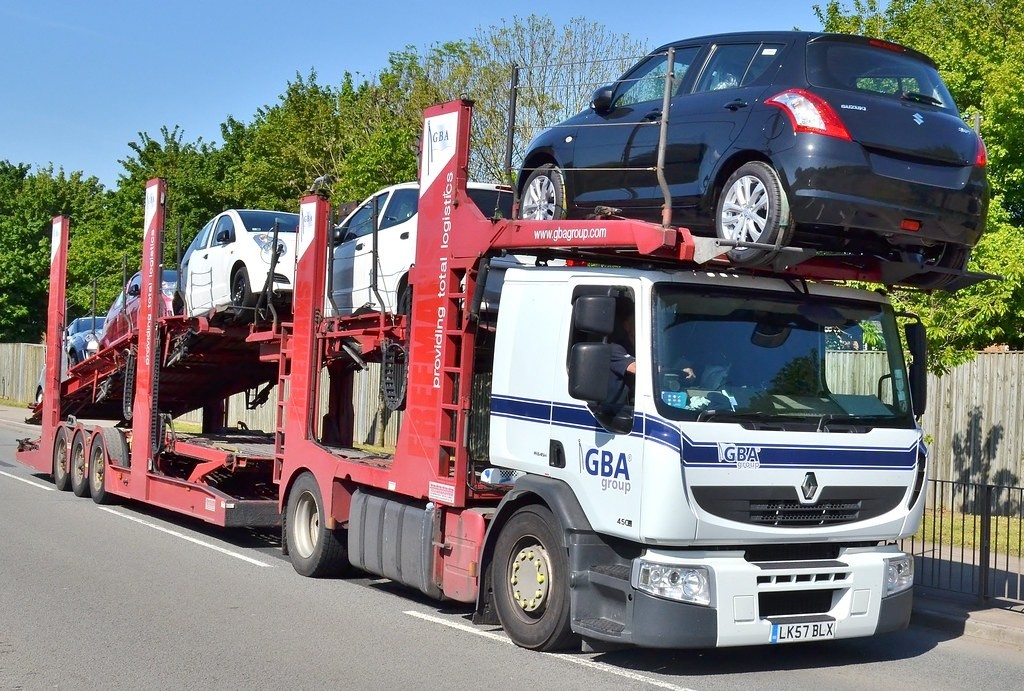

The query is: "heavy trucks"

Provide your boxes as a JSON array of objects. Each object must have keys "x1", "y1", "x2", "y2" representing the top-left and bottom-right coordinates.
[{"x1": 16, "y1": 99, "x2": 929, "y2": 653}]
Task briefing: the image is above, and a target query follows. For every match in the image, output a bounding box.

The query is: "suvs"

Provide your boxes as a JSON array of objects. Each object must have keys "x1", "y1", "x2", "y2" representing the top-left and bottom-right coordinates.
[{"x1": 517, "y1": 30, "x2": 990, "y2": 290}]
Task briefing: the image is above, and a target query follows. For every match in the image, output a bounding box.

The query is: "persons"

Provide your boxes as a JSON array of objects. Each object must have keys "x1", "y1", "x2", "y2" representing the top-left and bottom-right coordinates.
[{"x1": 609, "y1": 314, "x2": 695, "y2": 405}]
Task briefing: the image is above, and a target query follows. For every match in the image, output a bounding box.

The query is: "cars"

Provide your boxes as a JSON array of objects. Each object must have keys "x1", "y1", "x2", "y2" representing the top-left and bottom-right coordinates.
[
  {"x1": 98, "y1": 268, "x2": 177, "y2": 352},
  {"x1": 181, "y1": 209, "x2": 338, "y2": 319},
  {"x1": 31, "y1": 317, "x2": 105, "y2": 405},
  {"x1": 325, "y1": 180, "x2": 567, "y2": 315}
]
